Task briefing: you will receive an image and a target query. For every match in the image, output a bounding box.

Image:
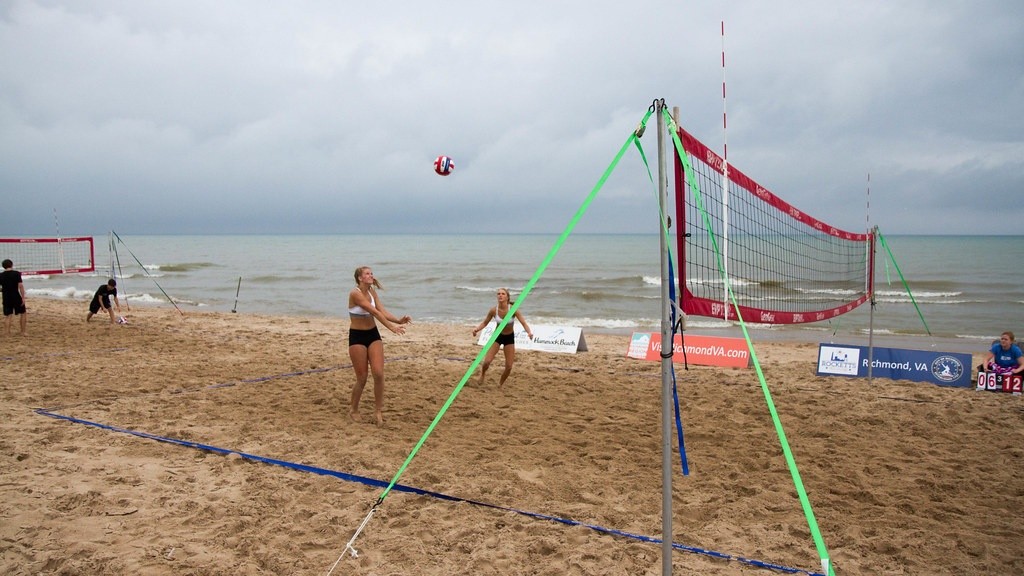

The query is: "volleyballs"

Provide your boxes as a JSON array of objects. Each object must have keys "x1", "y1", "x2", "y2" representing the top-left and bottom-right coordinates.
[
  {"x1": 118, "y1": 316, "x2": 128, "y2": 324},
  {"x1": 432, "y1": 155, "x2": 455, "y2": 176}
]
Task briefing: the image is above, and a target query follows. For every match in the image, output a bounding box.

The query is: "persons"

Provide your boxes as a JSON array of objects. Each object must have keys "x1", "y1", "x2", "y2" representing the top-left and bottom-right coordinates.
[
  {"x1": 87, "y1": 279, "x2": 121, "y2": 324},
  {"x1": 0, "y1": 259, "x2": 29, "y2": 337},
  {"x1": 977, "y1": 331, "x2": 1024, "y2": 382},
  {"x1": 473, "y1": 288, "x2": 533, "y2": 387},
  {"x1": 349, "y1": 266, "x2": 412, "y2": 424}
]
